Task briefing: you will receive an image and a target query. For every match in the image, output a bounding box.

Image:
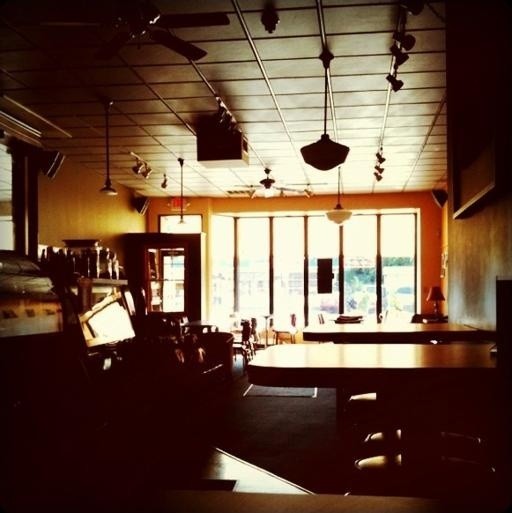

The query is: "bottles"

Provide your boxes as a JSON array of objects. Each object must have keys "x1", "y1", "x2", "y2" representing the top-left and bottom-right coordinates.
[{"x1": 41, "y1": 247, "x2": 120, "y2": 280}]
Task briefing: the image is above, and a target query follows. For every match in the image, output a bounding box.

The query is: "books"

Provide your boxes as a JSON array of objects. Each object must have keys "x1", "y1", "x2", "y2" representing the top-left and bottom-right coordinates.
[{"x1": 334, "y1": 314, "x2": 363, "y2": 323}]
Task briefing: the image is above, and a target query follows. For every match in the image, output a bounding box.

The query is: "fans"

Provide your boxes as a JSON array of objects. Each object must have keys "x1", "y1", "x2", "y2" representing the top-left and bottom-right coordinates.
[{"x1": 40, "y1": 1, "x2": 230, "y2": 63}]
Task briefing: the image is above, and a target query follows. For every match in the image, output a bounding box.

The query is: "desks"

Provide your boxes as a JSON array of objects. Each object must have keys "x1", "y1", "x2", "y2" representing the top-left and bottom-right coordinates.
[{"x1": 244, "y1": 319, "x2": 499, "y2": 489}]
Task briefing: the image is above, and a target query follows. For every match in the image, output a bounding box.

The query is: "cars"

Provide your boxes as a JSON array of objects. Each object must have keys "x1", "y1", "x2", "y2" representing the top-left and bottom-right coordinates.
[{"x1": 321, "y1": 269, "x2": 416, "y2": 319}]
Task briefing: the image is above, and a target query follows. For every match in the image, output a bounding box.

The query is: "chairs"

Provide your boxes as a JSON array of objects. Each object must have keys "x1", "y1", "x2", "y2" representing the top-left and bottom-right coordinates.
[{"x1": 197, "y1": 332, "x2": 234, "y2": 378}]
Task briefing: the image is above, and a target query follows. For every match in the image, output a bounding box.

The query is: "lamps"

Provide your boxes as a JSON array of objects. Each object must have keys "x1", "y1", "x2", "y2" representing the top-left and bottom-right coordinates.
[
  {"x1": 133, "y1": 193, "x2": 150, "y2": 215},
  {"x1": 385, "y1": 1, "x2": 425, "y2": 93},
  {"x1": 128, "y1": 153, "x2": 168, "y2": 189},
  {"x1": 373, "y1": 144, "x2": 387, "y2": 183},
  {"x1": 32, "y1": 146, "x2": 67, "y2": 178},
  {"x1": 425, "y1": 287, "x2": 446, "y2": 315},
  {"x1": 97, "y1": 95, "x2": 119, "y2": 197},
  {"x1": 302, "y1": 49, "x2": 355, "y2": 227},
  {"x1": 194, "y1": 102, "x2": 244, "y2": 161}
]
